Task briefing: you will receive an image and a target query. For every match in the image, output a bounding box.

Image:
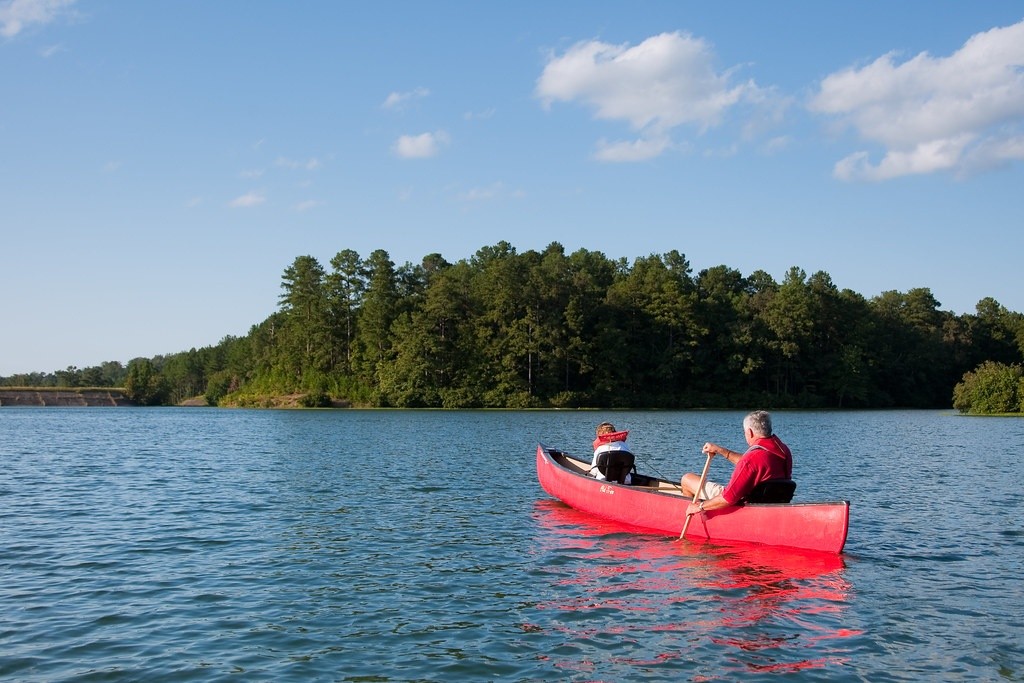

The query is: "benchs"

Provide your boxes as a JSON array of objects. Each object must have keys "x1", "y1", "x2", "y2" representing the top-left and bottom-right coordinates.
[{"x1": 625, "y1": 487, "x2": 682, "y2": 490}]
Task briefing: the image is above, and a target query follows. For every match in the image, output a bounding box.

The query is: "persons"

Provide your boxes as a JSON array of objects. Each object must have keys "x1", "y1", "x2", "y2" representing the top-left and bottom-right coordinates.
[
  {"x1": 681, "y1": 411, "x2": 793, "y2": 517},
  {"x1": 590, "y1": 423, "x2": 634, "y2": 485}
]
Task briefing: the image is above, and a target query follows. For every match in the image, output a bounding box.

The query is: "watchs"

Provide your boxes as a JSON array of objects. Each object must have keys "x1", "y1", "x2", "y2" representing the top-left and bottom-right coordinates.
[{"x1": 700, "y1": 501, "x2": 704, "y2": 511}]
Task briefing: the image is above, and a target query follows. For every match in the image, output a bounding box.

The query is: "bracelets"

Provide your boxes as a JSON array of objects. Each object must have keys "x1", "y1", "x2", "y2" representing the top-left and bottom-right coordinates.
[{"x1": 726, "y1": 451, "x2": 732, "y2": 459}]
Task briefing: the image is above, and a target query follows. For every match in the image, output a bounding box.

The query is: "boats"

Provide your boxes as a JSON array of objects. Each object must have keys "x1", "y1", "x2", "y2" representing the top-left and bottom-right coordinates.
[{"x1": 534, "y1": 441, "x2": 852, "y2": 554}]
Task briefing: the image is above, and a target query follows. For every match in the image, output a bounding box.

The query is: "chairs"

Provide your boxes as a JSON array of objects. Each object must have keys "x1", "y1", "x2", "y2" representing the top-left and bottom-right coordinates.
[
  {"x1": 584, "y1": 451, "x2": 639, "y2": 480},
  {"x1": 751, "y1": 479, "x2": 798, "y2": 506}
]
